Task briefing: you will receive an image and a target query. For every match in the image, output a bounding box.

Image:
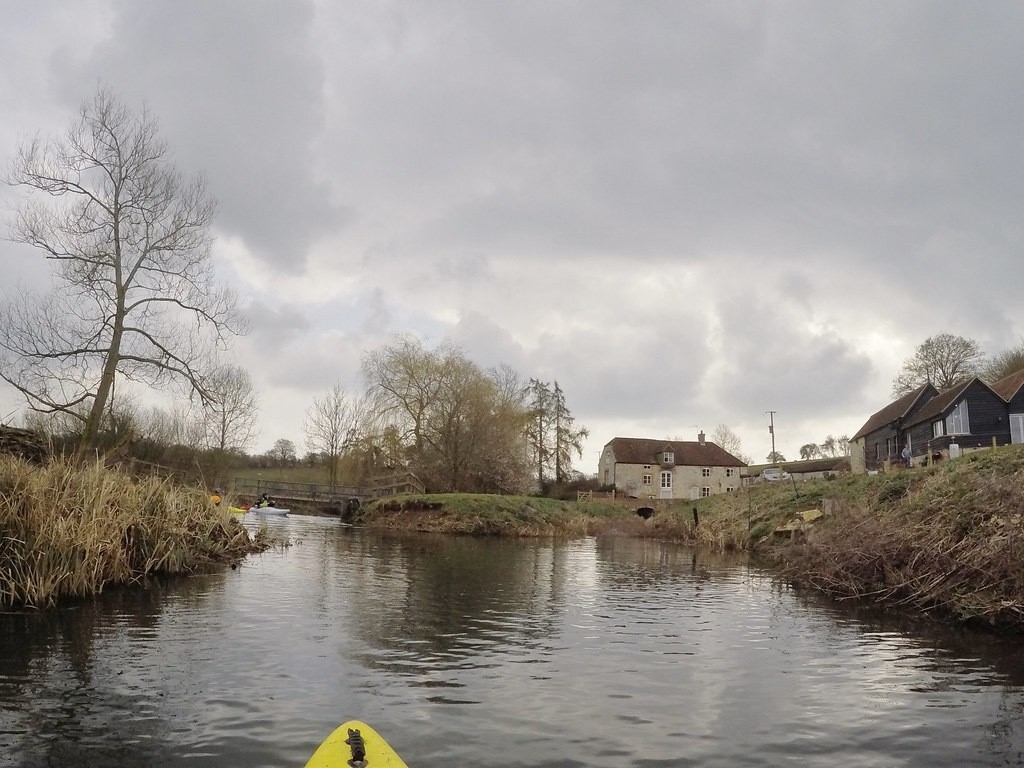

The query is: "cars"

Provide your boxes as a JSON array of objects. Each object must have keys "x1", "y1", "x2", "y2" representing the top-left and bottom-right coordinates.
[{"x1": 758, "y1": 467, "x2": 792, "y2": 482}]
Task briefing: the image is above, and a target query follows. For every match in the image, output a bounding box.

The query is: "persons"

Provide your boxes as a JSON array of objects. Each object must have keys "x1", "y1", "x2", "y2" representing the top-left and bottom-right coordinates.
[
  {"x1": 902, "y1": 444, "x2": 912, "y2": 469},
  {"x1": 253, "y1": 492, "x2": 276, "y2": 509},
  {"x1": 234, "y1": 494, "x2": 247, "y2": 506},
  {"x1": 209, "y1": 488, "x2": 222, "y2": 507}
]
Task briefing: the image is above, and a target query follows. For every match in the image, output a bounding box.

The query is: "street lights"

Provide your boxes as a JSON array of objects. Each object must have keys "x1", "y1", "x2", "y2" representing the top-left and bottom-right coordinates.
[{"x1": 765, "y1": 410, "x2": 778, "y2": 467}]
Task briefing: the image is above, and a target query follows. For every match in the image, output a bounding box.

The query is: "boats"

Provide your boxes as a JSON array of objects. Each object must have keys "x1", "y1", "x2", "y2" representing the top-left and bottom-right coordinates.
[
  {"x1": 220, "y1": 506, "x2": 247, "y2": 514},
  {"x1": 240, "y1": 504, "x2": 292, "y2": 516}
]
